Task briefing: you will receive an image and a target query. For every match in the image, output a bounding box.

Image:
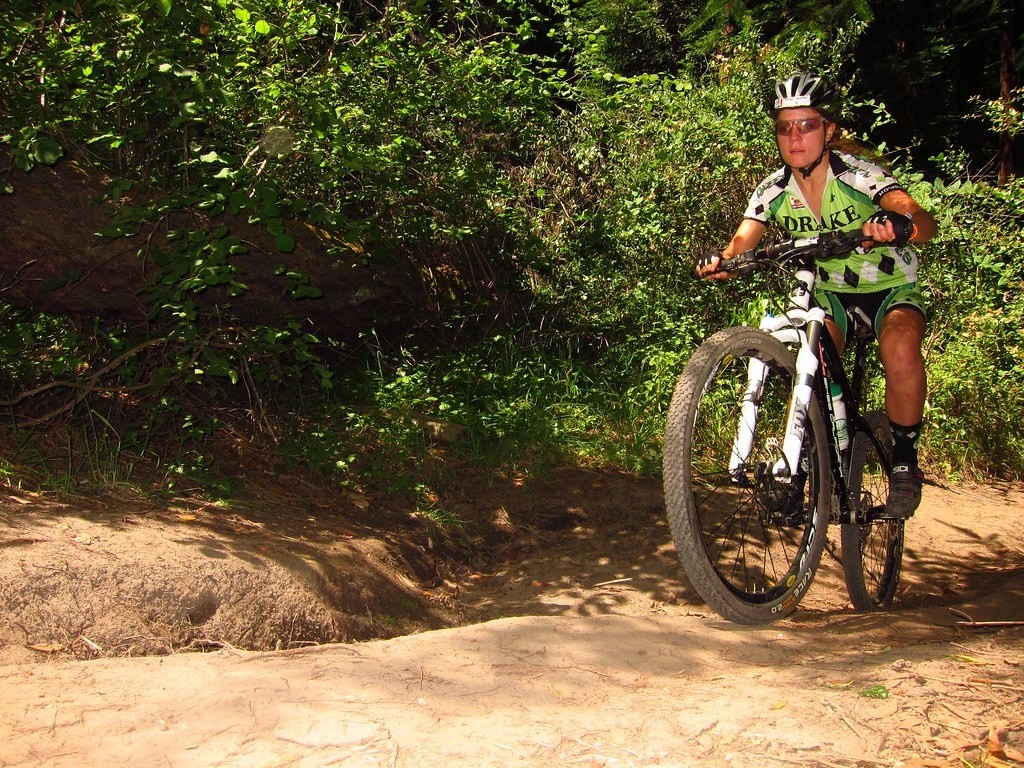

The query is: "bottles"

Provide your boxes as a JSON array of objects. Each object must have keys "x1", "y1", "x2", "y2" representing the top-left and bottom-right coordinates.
[{"x1": 826, "y1": 380, "x2": 849, "y2": 450}]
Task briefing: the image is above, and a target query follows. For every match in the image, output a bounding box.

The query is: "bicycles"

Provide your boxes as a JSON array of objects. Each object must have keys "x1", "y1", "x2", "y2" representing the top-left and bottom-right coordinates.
[{"x1": 661, "y1": 224, "x2": 907, "y2": 628}]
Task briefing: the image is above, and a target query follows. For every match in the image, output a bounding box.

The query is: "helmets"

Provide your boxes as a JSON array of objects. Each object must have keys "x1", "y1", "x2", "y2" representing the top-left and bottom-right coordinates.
[{"x1": 772, "y1": 73, "x2": 843, "y2": 122}]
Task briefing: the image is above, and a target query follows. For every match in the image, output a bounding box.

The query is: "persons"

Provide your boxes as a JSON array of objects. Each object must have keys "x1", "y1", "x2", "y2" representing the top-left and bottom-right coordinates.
[{"x1": 695, "y1": 71, "x2": 938, "y2": 517}]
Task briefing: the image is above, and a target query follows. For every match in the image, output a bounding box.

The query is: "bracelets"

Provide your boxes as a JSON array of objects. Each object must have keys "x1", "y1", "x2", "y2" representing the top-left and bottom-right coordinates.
[{"x1": 909, "y1": 224, "x2": 918, "y2": 240}]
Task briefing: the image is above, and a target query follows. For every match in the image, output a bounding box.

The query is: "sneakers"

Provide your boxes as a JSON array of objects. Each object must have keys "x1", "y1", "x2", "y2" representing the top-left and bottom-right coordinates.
[
  {"x1": 754, "y1": 489, "x2": 804, "y2": 515},
  {"x1": 886, "y1": 462, "x2": 923, "y2": 518}
]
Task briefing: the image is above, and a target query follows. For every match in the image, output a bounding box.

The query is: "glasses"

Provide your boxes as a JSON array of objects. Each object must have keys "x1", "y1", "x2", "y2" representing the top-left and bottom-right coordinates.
[{"x1": 773, "y1": 116, "x2": 831, "y2": 136}]
